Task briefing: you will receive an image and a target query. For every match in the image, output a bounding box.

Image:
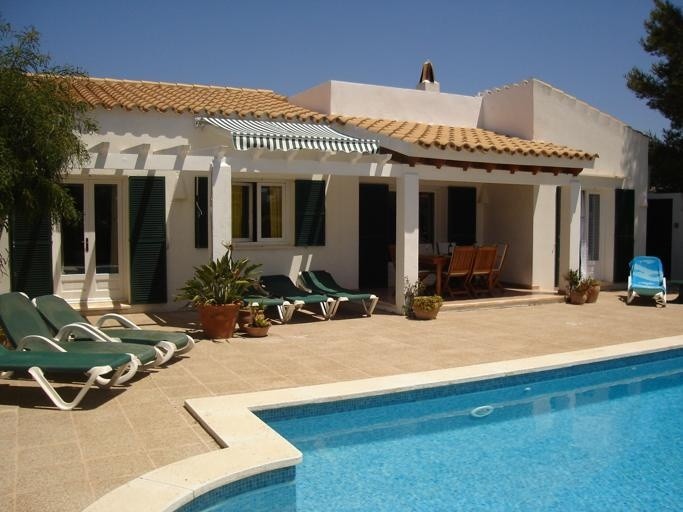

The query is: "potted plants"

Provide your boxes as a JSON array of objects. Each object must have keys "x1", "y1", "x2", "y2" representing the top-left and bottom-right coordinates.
[
  {"x1": 239, "y1": 300, "x2": 274, "y2": 338},
  {"x1": 584, "y1": 277, "x2": 602, "y2": 303},
  {"x1": 172, "y1": 238, "x2": 268, "y2": 343},
  {"x1": 399, "y1": 276, "x2": 444, "y2": 321},
  {"x1": 562, "y1": 269, "x2": 593, "y2": 304}
]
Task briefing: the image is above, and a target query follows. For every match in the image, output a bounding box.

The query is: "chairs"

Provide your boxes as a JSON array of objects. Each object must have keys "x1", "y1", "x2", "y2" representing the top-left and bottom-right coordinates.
[
  {"x1": 258, "y1": 274, "x2": 330, "y2": 324},
  {"x1": 624, "y1": 254, "x2": 668, "y2": 308},
  {"x1": 236, "y1": 276, "x2": 289, "y2": 325},
  {"x1": 0, "y1": 284, "x2": 163, "y2": 393},
  {"x1": 30, "y1": 290, "x2": 194, "y2": 367},
  {"x1": 389, "y1": 238, "x2": 510, "y2": 300},
  {"x1": 1, "y1": 336, "x2": 133, "y2": 413},
  {"x1": 296, "y1": 270, "x2": 380, "y2": 320}
]
